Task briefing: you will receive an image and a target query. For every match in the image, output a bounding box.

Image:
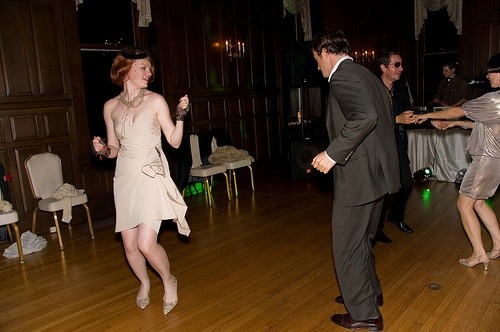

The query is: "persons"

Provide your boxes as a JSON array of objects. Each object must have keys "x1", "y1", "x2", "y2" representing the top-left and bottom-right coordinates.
[
  {"x1": 311, "y1": 24, "x2": 500, "y2": 331},
  {"x1": 90, "y1": 46, "x2": 195, "y2": 318}
]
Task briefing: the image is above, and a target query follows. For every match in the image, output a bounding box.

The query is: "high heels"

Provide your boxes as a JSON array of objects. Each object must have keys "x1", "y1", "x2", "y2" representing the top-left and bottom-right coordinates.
[
  {"x1": 460, "y1": 254, "x2": 490, "y2": 271},
  {"x1": 136, "y1": 281, "x2": 150, "y2": 310},
  {"x1": 486, "y1": 249, "x2": 500, "y2": 259},
  {"x1": 162, "y1": 277, "x2": 178, "y2": 315}
]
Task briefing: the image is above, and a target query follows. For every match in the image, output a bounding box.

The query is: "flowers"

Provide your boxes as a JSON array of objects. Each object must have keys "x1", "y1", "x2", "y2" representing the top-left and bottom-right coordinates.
[{"x1": 3, "y1": 174, "x2": 12, "y2": 182}]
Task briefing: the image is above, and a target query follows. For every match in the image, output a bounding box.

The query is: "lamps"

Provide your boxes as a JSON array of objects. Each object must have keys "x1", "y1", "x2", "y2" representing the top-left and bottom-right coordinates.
[
  {"x1": 225, "y1": 37, "x2": 246, "y2": 61},
  {"x1": 354, "y1": 49, "x2": 378, "y2": 68}
]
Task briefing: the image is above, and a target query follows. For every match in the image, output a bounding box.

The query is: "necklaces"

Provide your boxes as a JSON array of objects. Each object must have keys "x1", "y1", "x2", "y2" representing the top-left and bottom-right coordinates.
[{"x1": 117, "y1": 87, "x2": 143, "y2": 133}]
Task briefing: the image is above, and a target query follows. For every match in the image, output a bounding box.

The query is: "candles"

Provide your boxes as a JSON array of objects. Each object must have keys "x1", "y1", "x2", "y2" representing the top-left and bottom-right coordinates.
[{"x1": 298, "y1": 88, "x2": 302, "y2": 110}]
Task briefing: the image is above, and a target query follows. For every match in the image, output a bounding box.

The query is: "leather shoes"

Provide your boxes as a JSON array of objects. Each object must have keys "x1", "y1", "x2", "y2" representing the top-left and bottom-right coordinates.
[
  {"x1": 331, "y1": 313, "x2": 383, "y2": 331},
  {"x1": 335, "y1": 292, "x2": 384, "y2": 306},
  {"x1": 375, "y1": 231, "x2": 393, "y2": 243},
  {"x1": 387, "y1": 212, "x2": 413, "y2": 233}
]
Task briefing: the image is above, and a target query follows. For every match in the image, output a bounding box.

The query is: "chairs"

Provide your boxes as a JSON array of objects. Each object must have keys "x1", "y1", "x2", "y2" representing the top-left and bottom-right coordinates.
[
  {"x1": 204, "y1": 128, "x2": 255, "y2": 197},
  {"x1": 24, "y1": 152, "x2": 95, "y2": 249},
  {"x1": 0, "y1": 165, "x2": 25, "y2": 264},
  {"x1": 176, "y1": 134, "x2": 231, "y2": 206}
]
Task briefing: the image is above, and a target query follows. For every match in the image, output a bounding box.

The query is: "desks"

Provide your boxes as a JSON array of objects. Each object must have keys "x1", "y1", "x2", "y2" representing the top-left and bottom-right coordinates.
[{"x1": 406, "y1": 128, "x2": 471, "y2": 183}]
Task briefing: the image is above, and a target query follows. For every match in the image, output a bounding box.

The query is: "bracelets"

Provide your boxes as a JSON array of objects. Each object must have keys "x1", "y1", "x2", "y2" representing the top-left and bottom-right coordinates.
[
  {"x1": 105, "y1": 147, "x2": 111, "y2": 158},
  {"x1": 463, "y1": 120, "x2": 467, "y2": 130},
  {"x1": 176, "y1": 115, "x2": 184, "y2": 121},
  {"x1": 427, "y1": 113, "x2": 430, "y2": 117}
]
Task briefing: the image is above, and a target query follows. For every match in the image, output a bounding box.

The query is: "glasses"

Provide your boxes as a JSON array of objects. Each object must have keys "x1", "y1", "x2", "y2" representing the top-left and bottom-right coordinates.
[
  {"x1": 388, "y1": 62, "x2": 405, "y2": 68},
  {"x1": 443, "y1": 68, "x2": 449, "y2": 72}
]
